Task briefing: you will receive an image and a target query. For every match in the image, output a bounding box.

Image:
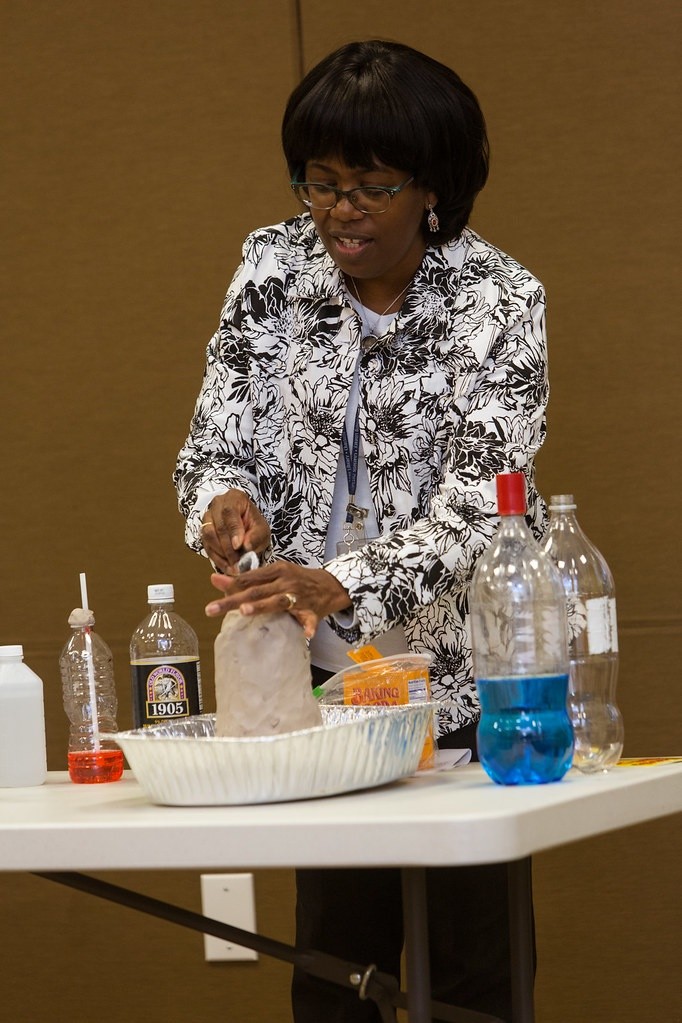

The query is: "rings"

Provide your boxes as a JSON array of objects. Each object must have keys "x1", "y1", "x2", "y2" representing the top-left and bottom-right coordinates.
[
  {"x1": 199, "y1": 522, "x2": 212, "y2": 532},
  {"x1": 284, "y1": 593, "x2": 296, "y2": 610}
]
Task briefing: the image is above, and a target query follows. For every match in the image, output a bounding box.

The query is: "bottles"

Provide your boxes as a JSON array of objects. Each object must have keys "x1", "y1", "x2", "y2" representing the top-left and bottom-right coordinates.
[
  {"x1": 129, "y1": 583, "x2": 204, "y2": 729},
  {"x1": 467, "y1": 474, "x2": 576, "y2": 786},
  {"x1": 60, "y1": 608, "x2": 124, "y2": 784},
  {"x1": 539, "y1": 494, "x2": 625, "y2": 774}
]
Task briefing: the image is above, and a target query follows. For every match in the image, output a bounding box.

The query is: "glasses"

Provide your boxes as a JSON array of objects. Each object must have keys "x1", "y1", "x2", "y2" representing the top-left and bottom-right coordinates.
[{"x1": 289, "y1": 161, "x2": 414, "y2": 214}]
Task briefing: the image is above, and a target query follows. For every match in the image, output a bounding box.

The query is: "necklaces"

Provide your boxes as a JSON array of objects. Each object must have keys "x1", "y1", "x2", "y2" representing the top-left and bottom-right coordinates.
[{"x1": 351, "y1": 275, "x2": 413, "y2": 355}]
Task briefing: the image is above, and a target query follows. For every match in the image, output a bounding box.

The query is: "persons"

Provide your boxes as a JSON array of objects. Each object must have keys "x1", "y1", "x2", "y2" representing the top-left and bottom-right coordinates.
[{"x1": 173, "y1": 40, "x2": 550, "y2": 1023}]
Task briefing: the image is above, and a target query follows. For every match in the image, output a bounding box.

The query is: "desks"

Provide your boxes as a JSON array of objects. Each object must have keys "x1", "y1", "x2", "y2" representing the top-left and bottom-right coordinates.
[{"x1": 0, "y1": 756, "x2": 682, "y2": 1023}]
